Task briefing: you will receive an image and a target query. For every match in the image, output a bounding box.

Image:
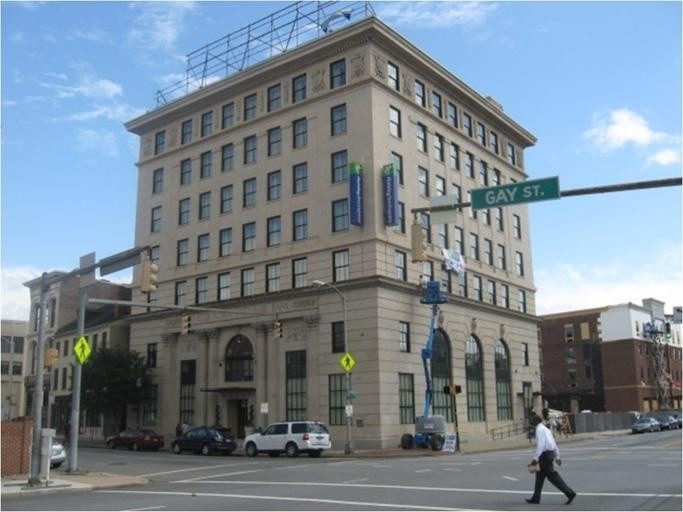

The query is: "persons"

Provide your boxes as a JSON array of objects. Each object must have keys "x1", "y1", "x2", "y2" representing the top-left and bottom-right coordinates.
[
  {"x1": 417, "y1": 274, "x2": 428, "y2": 297},
  {"x1": 176, "y1": 419, "x2": 190, "y2": 437},
  {"x1": 524, "y1": 409, "x2": 572, "y2": 443},
  {"x1": 525, "y1": 414, "x2": 577, "y2": 505}
]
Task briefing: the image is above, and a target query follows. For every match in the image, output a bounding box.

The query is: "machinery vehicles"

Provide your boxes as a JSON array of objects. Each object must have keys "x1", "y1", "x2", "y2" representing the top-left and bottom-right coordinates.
[{"x1": 401, "y1": 281, "x2": 448, "y2": 452}]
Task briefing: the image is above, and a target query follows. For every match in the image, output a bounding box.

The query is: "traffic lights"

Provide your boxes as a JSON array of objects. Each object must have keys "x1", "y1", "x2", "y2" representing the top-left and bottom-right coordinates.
[
  {"x1": 151, "y1": 262, "x2": 160, "y2": 292},
  {"x1": 277, "y1": 322, "x2": 284, "y2": 339},
  {"x1": 666, "y1": 323, "x2": 672, "y2": 338}
]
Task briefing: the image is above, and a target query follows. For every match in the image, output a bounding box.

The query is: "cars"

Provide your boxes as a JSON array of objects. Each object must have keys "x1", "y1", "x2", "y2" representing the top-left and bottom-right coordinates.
[
  {"x1": 105, "y1": 426, "x2": 165, "y2": 450},
  {"x1": 244, "y1": 421, "x2": 332, "y2": 457},
  {"x1": 170, "y1": 427, "x2": 237, "y2": 455},
  {"x1": 52, "y1": 439, "x2": 66, "y2": 467},
  {"x1": 632, "y1": 416, "x2": 682, "y2": 434}
]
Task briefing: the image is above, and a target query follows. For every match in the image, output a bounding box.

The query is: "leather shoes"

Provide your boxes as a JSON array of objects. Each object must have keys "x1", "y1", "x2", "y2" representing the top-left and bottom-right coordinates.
[
  {"x1": 563, "y1": 494, "x2": 577, "y2": 506},
  {"x1": 526, "y1": 498, "x2": 540, "y2": 505}
]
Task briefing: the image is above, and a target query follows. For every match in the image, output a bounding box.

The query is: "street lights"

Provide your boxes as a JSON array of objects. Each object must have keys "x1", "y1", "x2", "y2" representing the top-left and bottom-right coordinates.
[
  {"x1": 313, "y1": 279, "x2": 353, "y2": 454},
  {"x1": 184, "y1": 316, "x2": 191, "y2": 334}
]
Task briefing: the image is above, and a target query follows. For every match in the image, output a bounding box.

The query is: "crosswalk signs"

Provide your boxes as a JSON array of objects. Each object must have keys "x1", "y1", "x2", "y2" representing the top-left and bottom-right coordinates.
[
  {"x1": 75, "y1": 338, "x2": 91, "y2": 364},
  {"x1": 341, "y1": 353, "x2": 357, "y2": 371}
]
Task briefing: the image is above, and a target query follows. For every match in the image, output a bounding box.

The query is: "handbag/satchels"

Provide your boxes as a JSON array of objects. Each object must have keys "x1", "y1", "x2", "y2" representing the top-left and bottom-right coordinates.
[{"x1": 528, "y1": 463, "x2": 539, "y2": 473}]
